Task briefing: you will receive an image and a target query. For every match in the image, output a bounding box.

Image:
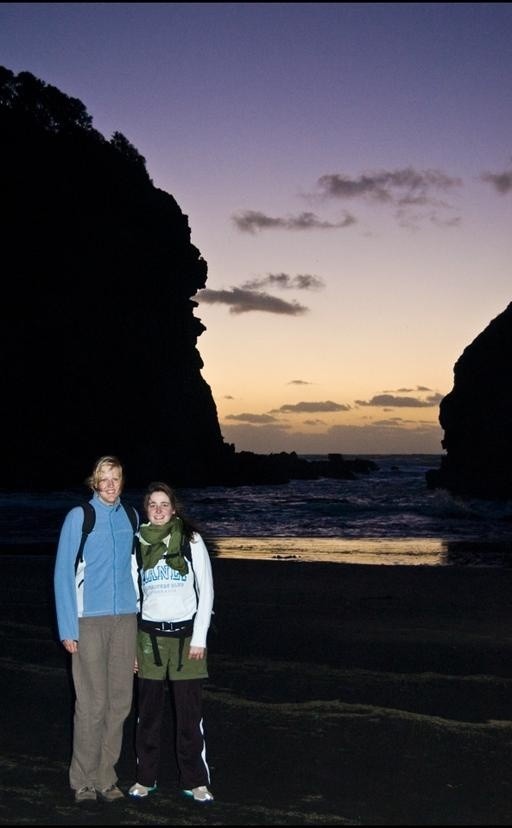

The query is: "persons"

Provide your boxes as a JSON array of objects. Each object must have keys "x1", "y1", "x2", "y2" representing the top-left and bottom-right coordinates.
[
  {"x1": 53, "y1": 456, "x2": 140, "y2": 805},
  {"x1": 129, "y1": 481, "x2": 215, "y2": 805}
]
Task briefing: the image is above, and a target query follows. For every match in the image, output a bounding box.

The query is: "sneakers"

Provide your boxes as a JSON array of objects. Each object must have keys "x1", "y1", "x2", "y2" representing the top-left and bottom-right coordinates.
[
  {"x1": 74, "y1": 785, "x2": 97, "y2": 802},
  {"x1": 101, "y1": 784, "x2": 125, "y2": 803},
  {"x1": 128, "y1": 779, "x2": 158, "y2": 798},
  {"x1": 184, "y1": 786, "x2": 214, "y2": 803}
]
